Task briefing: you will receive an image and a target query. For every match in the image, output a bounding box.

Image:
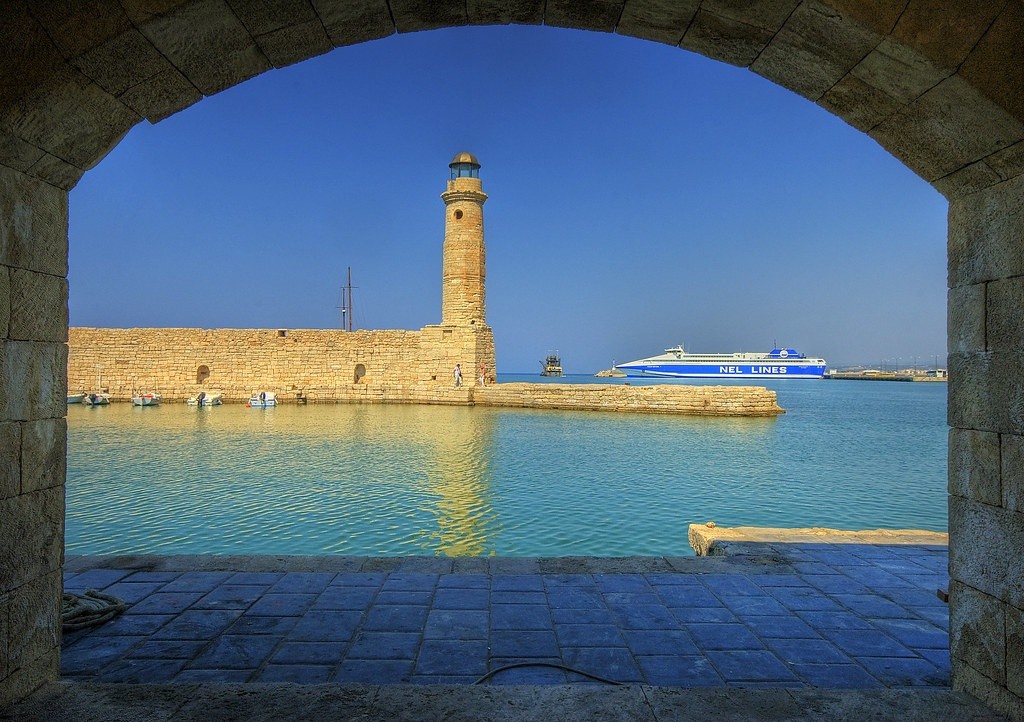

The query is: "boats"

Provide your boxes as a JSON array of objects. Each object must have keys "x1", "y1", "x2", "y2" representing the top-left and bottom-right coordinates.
[
  {"x1": 186, "y1": 390, "x2": 223, "y2": 406},
  {"x1": 538, "y1": 350, "x2": 566, "y2": 377},
  {"x1": 129, "y1": 393, "x2": 162, "y2": 406},
  {"x1": 248, "y1": 390, "x2": 279, "y2": 408},
  {"x1": 67, "y1": 394, "x2": 84, "y2": 403},
  {"x1": 614, "y1": 338, "x2": 828, "y2": 381},
  {"x1": 84, "y1": 392, "x2": 110, "y2": 404}
]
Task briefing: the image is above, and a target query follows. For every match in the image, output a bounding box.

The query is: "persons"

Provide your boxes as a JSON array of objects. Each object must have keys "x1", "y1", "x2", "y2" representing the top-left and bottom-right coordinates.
[
  {"x1": 476, "y1": 363, "x2": 485, "y2": 387},
  {"x1": 452, "y1": 363, "x2": 463, "y2": 387}
]
